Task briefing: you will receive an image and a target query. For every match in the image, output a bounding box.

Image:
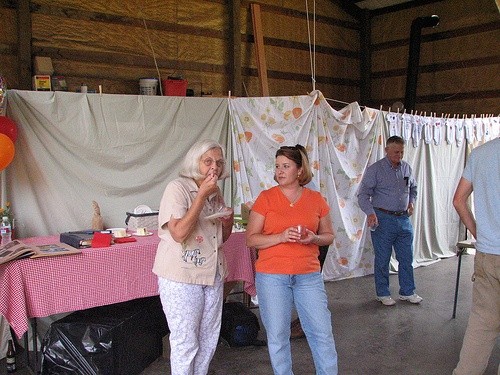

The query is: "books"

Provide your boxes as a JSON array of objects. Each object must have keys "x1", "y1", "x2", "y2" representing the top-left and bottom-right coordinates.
[
  {"x1": 0, "y1": 240, "x2": 82, "y2": 265},
  {"x1": 60, "y1": 233, "x2": 114, "y2": 248}
]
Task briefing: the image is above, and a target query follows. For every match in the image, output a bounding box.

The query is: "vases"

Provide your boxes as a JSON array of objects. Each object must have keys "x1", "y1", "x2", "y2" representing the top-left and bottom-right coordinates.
[{"x1": 10, "y1": 229, "x2": 15, "y2": 241}]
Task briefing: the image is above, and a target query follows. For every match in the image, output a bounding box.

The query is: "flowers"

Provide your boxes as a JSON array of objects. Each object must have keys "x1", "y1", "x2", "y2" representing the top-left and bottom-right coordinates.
[{"x1": 0, "y1": 201, "x2": 15, "y2": 229}]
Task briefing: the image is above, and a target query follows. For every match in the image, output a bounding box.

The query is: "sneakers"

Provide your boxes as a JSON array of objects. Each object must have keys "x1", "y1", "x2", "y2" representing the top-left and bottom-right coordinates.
[
  {"x1": 376, "y1": 295, "x2": 396, "y2": 305},
  {"x1": 398, "y1": 292, "x2": 423, "y2": 303}
]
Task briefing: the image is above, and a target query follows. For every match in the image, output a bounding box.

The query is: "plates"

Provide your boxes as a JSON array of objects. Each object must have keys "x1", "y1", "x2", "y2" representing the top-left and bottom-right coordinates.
[
  {"x1": 203, "y1": 210, "x2": 233, "y2": 221},
  {"x1": 113, "y1": 233, "x2": 131, "y2": 238},
  {"x1": 132, "y1": 230, "x2": 154, "y2": 237},
  {"x1": 134, "y1": 205, "x2": 152, "y2": 214}
]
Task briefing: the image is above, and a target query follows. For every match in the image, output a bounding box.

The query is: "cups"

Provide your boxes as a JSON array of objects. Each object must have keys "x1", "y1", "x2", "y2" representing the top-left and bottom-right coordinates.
[
  {"x1": 293, "y1": 225, "x2": 307, "y2": 241},
  {"x1": 368, "y1": 221, "x2": 378, "y2": 231}
]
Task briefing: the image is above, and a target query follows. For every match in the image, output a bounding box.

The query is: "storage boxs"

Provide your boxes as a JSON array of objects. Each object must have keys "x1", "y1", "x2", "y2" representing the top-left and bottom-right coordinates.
[
  {"x1": 161, "y1": 79, "x2": 188, "y2": 96},
  {"x1": 50, "y1": 305, "x2": 164, "y2": 373},
  {"x1": 33, "y1": 56, "x2": 54, "y2": 75},
  {"x1": 31, "y1": 75, "x2": 51, "y2": 91}
]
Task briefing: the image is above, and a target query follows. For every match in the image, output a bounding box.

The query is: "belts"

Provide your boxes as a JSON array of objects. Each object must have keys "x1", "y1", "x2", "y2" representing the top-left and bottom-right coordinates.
[{"x1": 373, "y1": 207, "x2": 407, "y2": 217}]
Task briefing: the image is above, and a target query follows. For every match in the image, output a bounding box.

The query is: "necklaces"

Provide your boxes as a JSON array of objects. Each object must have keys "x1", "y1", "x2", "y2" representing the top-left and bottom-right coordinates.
[{"x1": 279, "y1": 185, "x2": 303, "y2": 207}]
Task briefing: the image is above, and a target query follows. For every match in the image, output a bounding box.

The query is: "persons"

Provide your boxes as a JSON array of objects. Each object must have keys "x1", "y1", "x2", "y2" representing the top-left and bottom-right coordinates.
[
  {"x1": 453, "y1": 138, "x2": 500, "y2": 375},
  {"x1": 358, "y1": 135, "x2": 423, "y2": 305},
  {"x1": 248, "y1": 144, "x2": 339, "y2": 375},
  {"x1": 152, "y1": 138, "x2": 234, "y2": 375}
]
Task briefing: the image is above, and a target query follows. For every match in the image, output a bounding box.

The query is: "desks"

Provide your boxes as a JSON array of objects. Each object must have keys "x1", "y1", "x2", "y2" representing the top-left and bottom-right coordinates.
[
  {"x1": 452, "y1": 238, "x2": 478, "y2": 319},
  {"x1": 0, "y1": 229, "x2": 259, "y2": 375}
]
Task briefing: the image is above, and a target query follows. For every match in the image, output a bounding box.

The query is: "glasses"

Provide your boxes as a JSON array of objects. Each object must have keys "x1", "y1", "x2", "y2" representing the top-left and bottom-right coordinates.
[{"x1": 200, "y1": 159, "x2": 225, "y2": 167}]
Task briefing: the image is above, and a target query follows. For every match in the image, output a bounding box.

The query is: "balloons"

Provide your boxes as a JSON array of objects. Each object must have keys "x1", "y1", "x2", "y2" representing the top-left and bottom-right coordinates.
[{"x1": 0, "y1": 116, "x2": 17, "y2": 169}]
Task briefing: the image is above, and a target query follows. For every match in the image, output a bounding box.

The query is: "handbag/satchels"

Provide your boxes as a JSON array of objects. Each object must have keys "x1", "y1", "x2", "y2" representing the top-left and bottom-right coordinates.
[{"x1": 223, "y1": 303, "x2": 267, "y2": 347}]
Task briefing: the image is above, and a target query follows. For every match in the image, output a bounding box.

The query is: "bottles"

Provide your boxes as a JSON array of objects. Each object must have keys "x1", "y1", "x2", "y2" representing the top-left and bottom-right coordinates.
[{"x1": 0, "y1": 217, "x2": 12, "y2": 246}]
